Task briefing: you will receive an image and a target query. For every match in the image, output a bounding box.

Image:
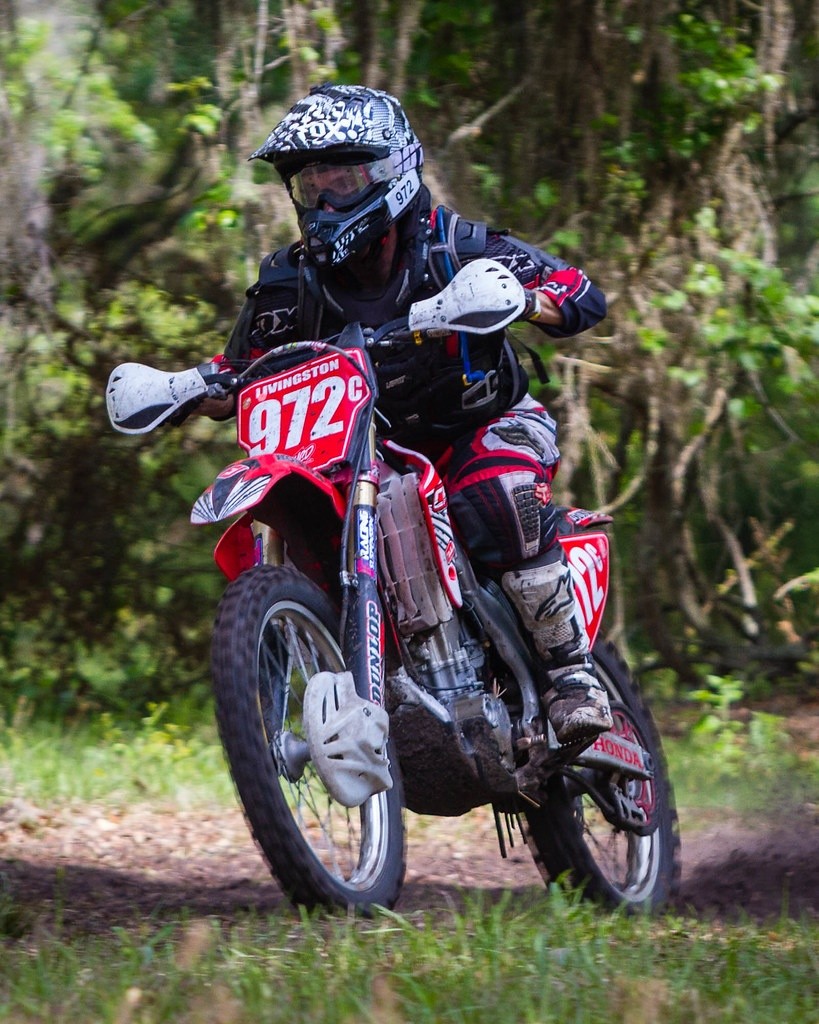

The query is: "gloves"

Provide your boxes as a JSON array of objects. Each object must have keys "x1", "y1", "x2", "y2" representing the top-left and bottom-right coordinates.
[{"x1": 514, "y1": 286, "x2": 540, "y2": 323}]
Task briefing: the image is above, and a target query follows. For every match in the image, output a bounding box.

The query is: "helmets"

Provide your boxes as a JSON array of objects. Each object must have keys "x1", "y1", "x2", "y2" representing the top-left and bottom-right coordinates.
[{"x1": 247, "y1": 84, "x2": 422, "y2": 270}]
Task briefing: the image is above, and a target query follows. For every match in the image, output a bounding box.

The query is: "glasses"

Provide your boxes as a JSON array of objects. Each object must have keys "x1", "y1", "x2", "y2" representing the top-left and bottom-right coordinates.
[{"x1": 289, "y1": 162, "x2": 373, "y2": 210}]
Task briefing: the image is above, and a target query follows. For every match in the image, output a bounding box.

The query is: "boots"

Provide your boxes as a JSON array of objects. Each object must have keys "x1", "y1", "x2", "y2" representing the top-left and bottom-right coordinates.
[{"x1": 492, "y1": 544, "x2": 613, "y2": 743}]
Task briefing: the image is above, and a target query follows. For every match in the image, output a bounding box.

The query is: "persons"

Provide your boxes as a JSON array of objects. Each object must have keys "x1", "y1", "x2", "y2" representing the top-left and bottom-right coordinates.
[{"x1": 191, "y1": 86, "x2": 614, "y2": 738}]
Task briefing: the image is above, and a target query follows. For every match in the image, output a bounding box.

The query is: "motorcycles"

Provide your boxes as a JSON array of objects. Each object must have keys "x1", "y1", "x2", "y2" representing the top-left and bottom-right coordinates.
[{"x1": 106, "y1": 257, "x2": 682, "y2": 921}]
